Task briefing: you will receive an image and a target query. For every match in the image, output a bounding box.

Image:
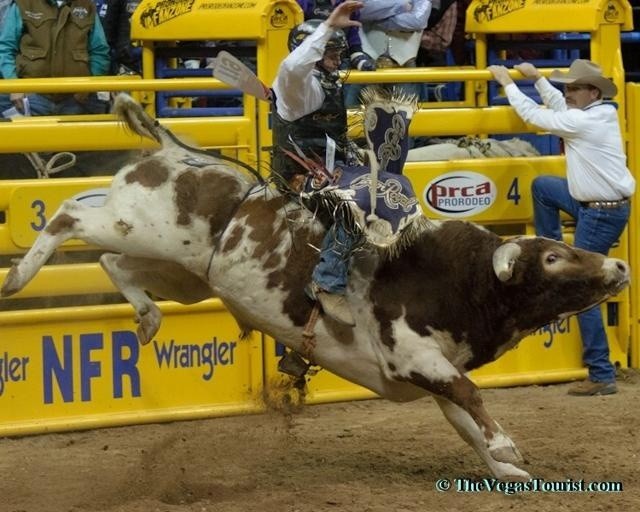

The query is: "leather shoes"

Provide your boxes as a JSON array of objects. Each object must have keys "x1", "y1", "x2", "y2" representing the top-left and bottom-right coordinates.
[
  {"x1": 567, "y1": 379, "x2": 618, "y2": 396},
  {"x1": 305, "y1": 281, "x2": 356, "y2": 328}
]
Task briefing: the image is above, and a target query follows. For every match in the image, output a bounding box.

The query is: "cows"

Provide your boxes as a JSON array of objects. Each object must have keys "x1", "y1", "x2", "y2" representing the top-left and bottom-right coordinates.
[{"x1": 0, "y1": 89, "x2": 633, "y2": 487}]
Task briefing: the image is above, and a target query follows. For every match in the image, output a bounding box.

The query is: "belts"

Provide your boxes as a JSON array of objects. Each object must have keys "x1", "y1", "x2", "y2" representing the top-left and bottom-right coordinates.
[{"x1": 584, "y1": 200, "x2": 626, "y2": 209}]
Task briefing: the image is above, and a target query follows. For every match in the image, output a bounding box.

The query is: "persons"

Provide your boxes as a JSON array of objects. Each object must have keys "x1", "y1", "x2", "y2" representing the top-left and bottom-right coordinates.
[
  {"x1": 343, "y1": 0, "x2": 434, "y2": 108},
  {"x1": 296, "y1": 0, "x2": 377, "y2": 73},
  {"x1": 487, "y1": 59, "x2": 637, "y2": 396},
  {"x1": 416, "y1": 0, "x2": 462, "y2": 101},
  {"x1": 268, "y1": 0, "x2": 388, "y2": 329},
  {"x1": 0, "y1": 0, "x2": 112, "y2": 118}
]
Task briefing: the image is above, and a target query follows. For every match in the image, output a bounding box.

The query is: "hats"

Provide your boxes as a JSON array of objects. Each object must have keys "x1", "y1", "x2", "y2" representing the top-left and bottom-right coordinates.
[{"x1": 550, "y1": 59, "x2": 617, "y2": 100}]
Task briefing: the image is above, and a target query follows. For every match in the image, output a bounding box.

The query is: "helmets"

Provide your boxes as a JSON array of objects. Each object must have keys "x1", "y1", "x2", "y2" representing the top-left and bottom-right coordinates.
[{"x1": 288, "y1": 18, "x2": 351, "y2": 81}]
